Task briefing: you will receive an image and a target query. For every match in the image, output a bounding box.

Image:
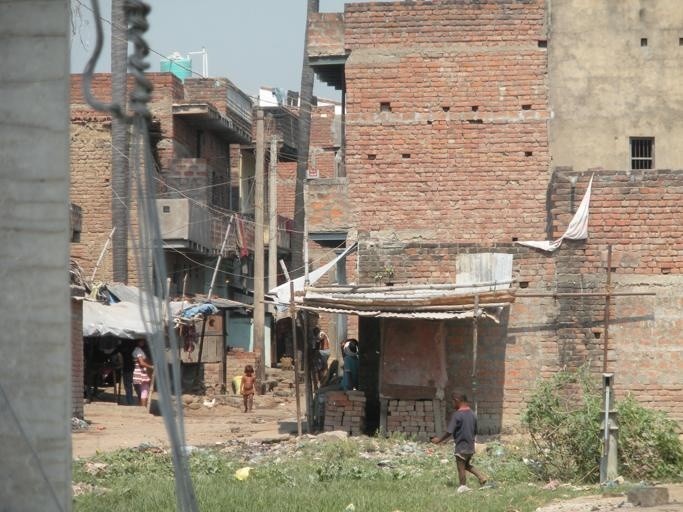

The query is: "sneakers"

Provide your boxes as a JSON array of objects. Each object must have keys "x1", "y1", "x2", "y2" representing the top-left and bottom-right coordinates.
[{"x1": 479, "y1": 478, "x2": 487, "y2": 487}]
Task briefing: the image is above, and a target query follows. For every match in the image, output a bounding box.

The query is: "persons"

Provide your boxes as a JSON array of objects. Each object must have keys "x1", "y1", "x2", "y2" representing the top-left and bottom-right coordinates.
[
  {"x1": 342, "y1": 341, "x2": 359, "y2": 391},
  {"x1": 132, "y1": 340, "x2": 154, "y2": 406},
  {"x1": 432, "y1": 391, "x2": 487, "y2": 494},
  {"x1": 240, "y1": 365, "x2": 258, "y2": 413},
  {"x1": 309, "y1": 328, "x2": 331, "y2": 373}
]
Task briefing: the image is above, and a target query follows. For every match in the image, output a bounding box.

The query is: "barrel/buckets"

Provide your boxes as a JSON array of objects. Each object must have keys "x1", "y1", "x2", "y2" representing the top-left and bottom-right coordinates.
[{"x1": 160, "y1": 59, "x2": 193, "y2": 84}]
